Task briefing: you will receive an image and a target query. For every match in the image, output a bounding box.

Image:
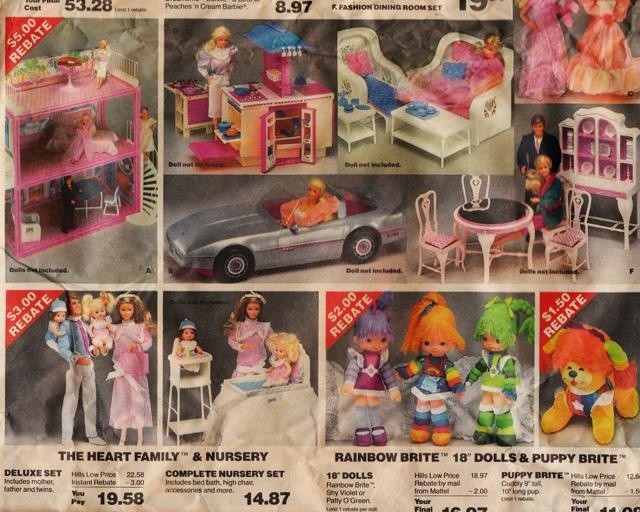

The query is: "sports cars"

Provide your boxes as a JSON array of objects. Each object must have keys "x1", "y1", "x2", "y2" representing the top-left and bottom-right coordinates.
[{"x1": 166, "y1": 177, "x2": 407, "y2": 283}]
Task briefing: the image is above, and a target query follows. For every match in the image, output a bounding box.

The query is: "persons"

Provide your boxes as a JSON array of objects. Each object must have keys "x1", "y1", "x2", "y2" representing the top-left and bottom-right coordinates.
[{"x1": 2, "y1": 2, "x2": 639, "y2": 449}]
